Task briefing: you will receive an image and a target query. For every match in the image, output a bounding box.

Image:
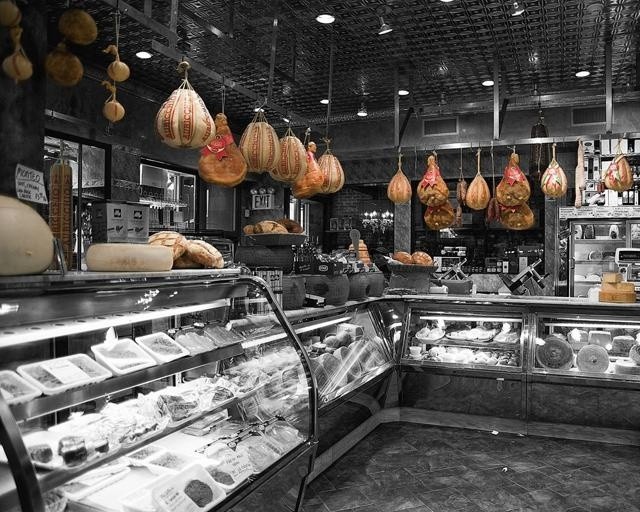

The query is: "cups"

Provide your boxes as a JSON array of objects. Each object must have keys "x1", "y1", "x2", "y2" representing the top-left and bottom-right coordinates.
[
  {"x1": 410, "y1": 346, "x2": 423, "y2": 355},
  {"x1": 429, "y1": 346, "x2": 517, "y2": 367}
]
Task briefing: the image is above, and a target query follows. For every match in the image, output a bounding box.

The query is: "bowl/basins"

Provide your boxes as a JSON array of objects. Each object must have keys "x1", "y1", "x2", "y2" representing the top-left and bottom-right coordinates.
[
  {"x1": 297, "y1": 359, "x2": 332, "y2": 396},
  {"x1": 319, "y1": 352, "x2": 348, "y2": 388},
  {"x1": 347, "y1": 340, "x2": 375, "y2": 372},
  {"x1": 332, "y1": 345, "x2": 362, "y2": 379}
]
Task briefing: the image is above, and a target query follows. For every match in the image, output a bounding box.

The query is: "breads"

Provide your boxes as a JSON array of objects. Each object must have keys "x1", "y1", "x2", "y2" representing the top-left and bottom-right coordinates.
[
  {"x1": 276, "y1": 219, "x2": 302, "y2": 233},
  {"x1": 253, "y1": 220, "x2": 289, "y2": 234},
  {"x1": 147, "y1": 229, "x2": 187, "y2": 261},
  {"x1": 243, "y1": 224, "x2": 254, "y2": 234},
  {"x1": 174, "y1": 253, "x2": 202, "y2": 268},
  {"x1": 392, "y1": 251, "x2": 415, "y2": 264},
  {"x1": 412, "y1": 251, "x2": 432, "y2": 265},
  {"x1": 186, "y1": 239, "x2": 225, "y2": 269}
]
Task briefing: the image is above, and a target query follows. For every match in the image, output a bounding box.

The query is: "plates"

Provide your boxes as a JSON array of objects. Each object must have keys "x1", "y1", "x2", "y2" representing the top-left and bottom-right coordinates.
[
  {"x1": 126, "y1": 444, "x2": 190, "y2": 476},
  {"x1": 165, "y1": 323, "x2": 219, "y2": 357},
  {"x1": 387, "y1": 263, "x2": 439, "y2": 273},
  {"x1": 120, "y1": 378, "x2": 244, "y2": 430},
  {"x1": 182, "y1": 410, "x2": 233, "y2": 438},
  {"x1": 47, "y1": 413, "x2": 167, "y2": 451},
  {"x1": 198, "y1": 318, "x2": 247, "y2": 349},
  {"x1": 135, "y1": 332, "x2": 190, "y2": 366},
  {"x1": 0, "y1": 429, "x2": 122, "y2": 475},
  {"x1": 256, "y1": 414, "x2": 306, "y2": 457},
  {"x1": 0, "y1": 369, "x2": 43, "y2": 406},
  {"x1": 90, "y1": 338, "x2": 157, "y2": 377},
  {"x1": 408, "y1": 353, "x2": 424, "y2": 359},
  {"x1": 493, "y1": 327, "x2": 521, "y2": 345},
  {"x1": 246, "y1": 233, "x2": 307, "y2": 249},
  {"x1": 16, "y1": 352, "x2": 113, "y2": 396},
  {"x1": 151, "y1": 464, "x2": 227, "y2": 512},
  {"x1": 445, "y1": 329, "x2": 500, "y2": 342},
  {"x1": 201, "y1": 445, "x2": 255, "y2": 489},
  {"x1": 415, "y1": 328, "x2": 446, "y2": 340},
  {"x1": 236, "y1": 434, "x2": 286, "y2": 474}
]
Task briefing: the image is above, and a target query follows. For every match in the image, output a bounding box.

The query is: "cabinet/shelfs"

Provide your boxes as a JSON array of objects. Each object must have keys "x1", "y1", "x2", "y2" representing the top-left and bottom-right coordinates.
[
  {"x1": 228, "y1": 295, "x2": 640, "y2": 488},
  {"x1": 0, "y1": 267, "x2": 315, "y2": 512}
]
